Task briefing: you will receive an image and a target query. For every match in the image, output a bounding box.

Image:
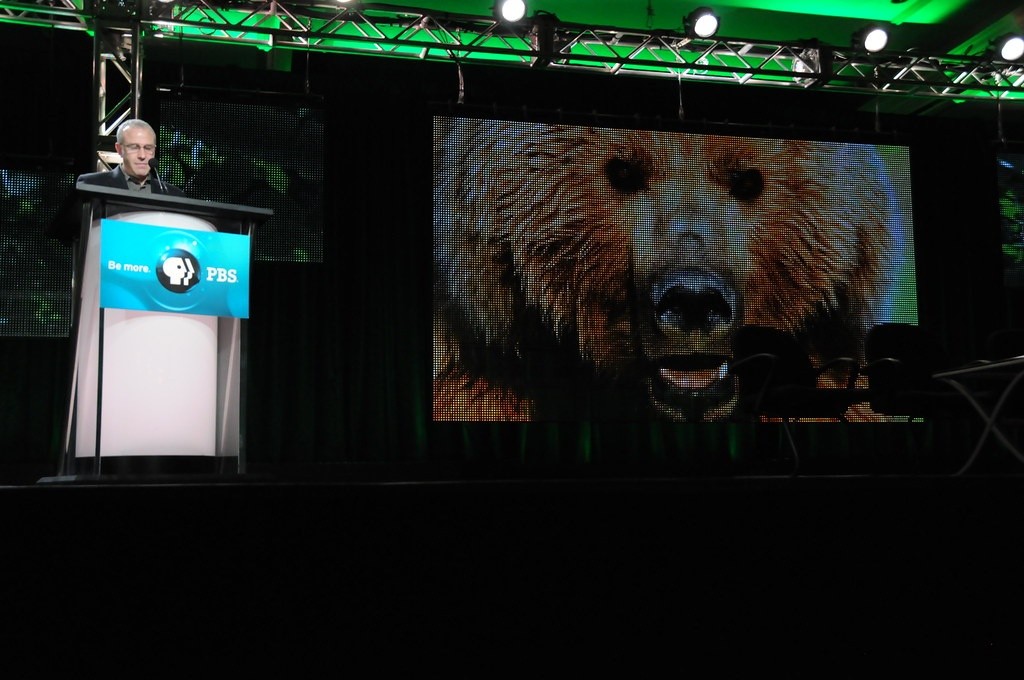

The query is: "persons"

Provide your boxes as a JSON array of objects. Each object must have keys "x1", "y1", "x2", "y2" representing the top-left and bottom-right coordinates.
[{"x1": 76, "y1": 119, "x2": 189, "y2": 198}]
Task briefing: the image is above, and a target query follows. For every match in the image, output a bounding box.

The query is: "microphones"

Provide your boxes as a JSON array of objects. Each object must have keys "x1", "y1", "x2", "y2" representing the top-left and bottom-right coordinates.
[{"x1": 149, "y1": 158, "x2": 166, "y2": 194}]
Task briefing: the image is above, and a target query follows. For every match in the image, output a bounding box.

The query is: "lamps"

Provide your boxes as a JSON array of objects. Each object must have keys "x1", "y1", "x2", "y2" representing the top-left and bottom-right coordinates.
[
  {"x1": 978, "y1": 32, "x2": 1024, "y2": 68},
  {"x1": 851, "y1": 24, "x2": 888, "y2": 52},
  {"x1": 682, "y1": 7, "x2": 720, "y2": 40}
]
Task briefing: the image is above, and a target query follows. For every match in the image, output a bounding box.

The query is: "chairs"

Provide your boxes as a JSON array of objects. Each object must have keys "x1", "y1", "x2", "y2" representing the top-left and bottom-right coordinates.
[{"x1": 730, "y1": 325, "x2": 1024, "y2": 479}]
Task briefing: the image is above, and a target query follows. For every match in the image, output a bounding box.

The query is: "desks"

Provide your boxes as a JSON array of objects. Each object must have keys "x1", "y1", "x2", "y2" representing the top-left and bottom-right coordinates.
[{"x1": 932, "y1": 354, "x2": 1024, "y2": 478}]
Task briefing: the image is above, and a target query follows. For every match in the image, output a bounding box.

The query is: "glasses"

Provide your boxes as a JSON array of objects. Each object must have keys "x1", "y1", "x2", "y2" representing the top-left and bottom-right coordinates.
[{"x1": 121, "y1": 144, "x2": 156, "y2": 155}]
